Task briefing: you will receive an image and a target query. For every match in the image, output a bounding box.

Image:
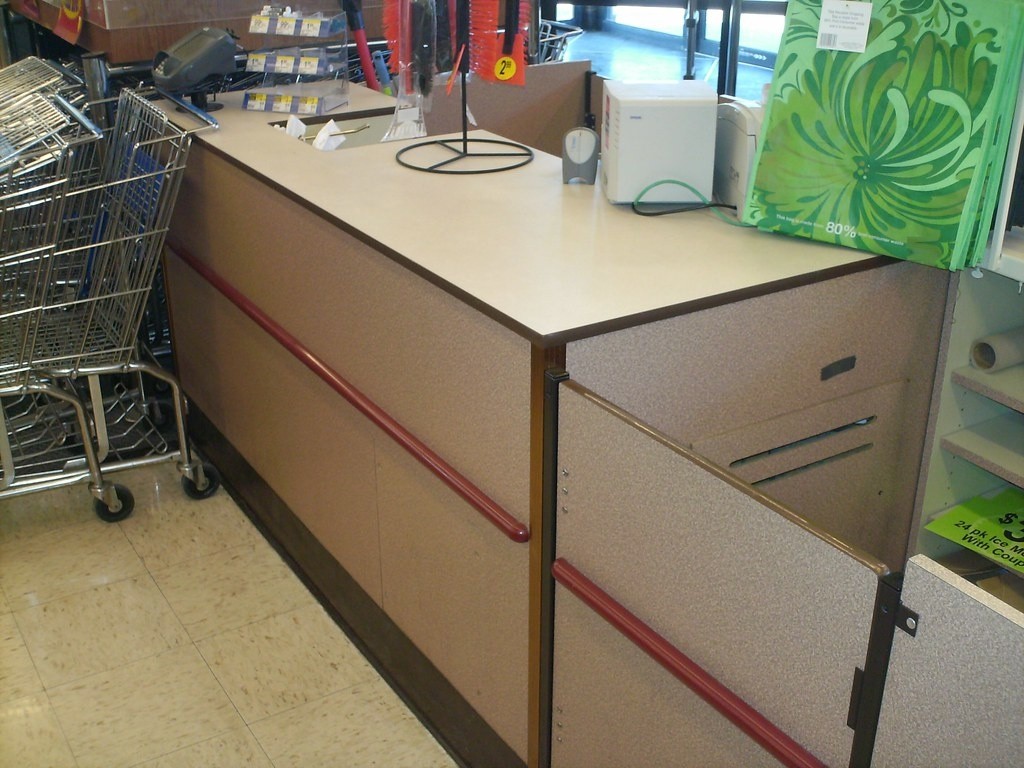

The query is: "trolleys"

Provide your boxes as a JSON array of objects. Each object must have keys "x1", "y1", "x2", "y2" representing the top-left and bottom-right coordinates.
[{"x1": 0, "y1": 18, "x2": 585, "y2": 523}]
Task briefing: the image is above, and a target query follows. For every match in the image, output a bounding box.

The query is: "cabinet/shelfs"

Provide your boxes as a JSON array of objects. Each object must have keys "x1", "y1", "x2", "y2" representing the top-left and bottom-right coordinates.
[
  {"x1": 900, "y1": 226, "x2": 1023, "y2": 616},
  {"x1": 144, "y1": 79, "x2": 951, "y2": 768}
]
